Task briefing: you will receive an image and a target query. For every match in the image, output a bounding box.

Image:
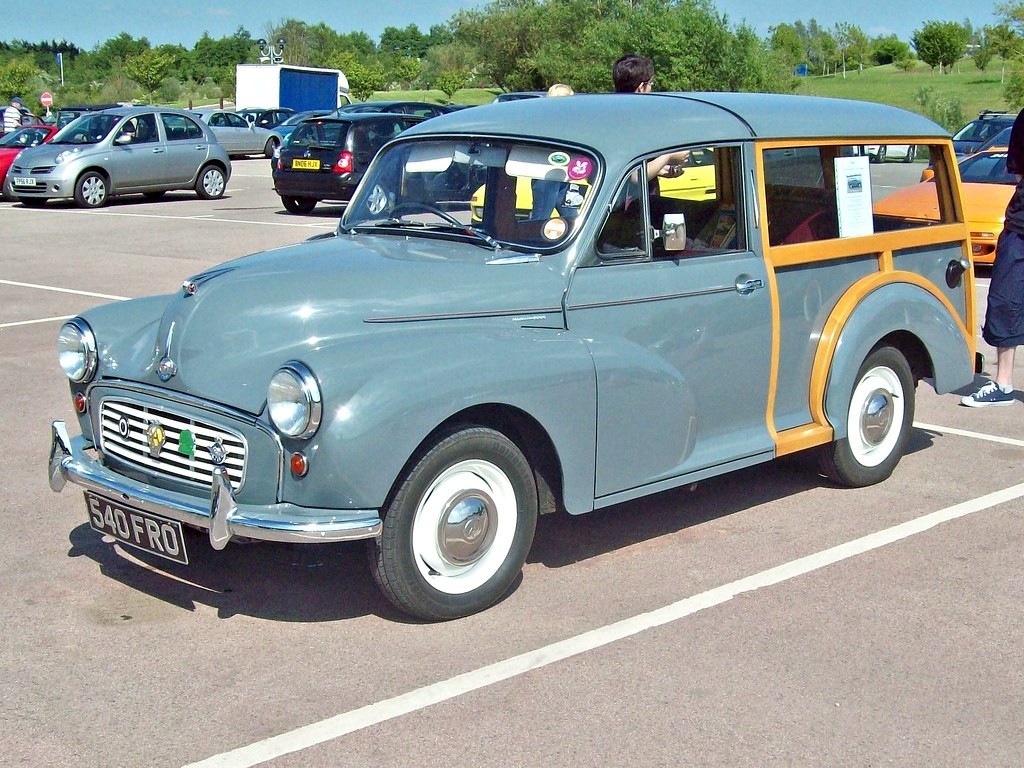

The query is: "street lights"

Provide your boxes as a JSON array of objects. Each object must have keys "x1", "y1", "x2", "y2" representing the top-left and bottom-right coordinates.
[{"x1": 257, "y1": 37, "x2": 286, "y2": 65}]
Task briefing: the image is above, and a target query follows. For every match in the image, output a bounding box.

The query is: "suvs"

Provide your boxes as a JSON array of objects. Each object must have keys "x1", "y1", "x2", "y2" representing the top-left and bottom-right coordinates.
[{"x1": 953, "y1": 109, "x2": 1019, "y2": 164}]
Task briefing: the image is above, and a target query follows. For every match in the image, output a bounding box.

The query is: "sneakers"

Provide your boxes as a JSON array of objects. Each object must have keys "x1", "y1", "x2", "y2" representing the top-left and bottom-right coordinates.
[{"x1": 961, "y1": 381, "x2": 1015, "y2": 407}]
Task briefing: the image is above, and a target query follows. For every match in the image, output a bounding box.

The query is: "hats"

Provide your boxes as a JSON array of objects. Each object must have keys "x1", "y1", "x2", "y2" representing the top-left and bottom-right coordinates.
[{"x1": 11, "y1": 97, "x2": 23, "y2": 106}]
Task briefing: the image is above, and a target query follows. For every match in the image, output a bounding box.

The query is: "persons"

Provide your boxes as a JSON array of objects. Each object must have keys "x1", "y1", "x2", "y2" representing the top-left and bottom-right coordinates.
[
  {"x1": 4, "y1": 97, "x2": 23, "y2": 135},
  {"x1": 588, "y1": 56, "x2": 690, "y2": 210},
  {"x1": 529, "y1": 84, "x2": 578, "y2": 219},
  {"x1": 963, "y1": 107, "x2": 1024, "y2": 407}
]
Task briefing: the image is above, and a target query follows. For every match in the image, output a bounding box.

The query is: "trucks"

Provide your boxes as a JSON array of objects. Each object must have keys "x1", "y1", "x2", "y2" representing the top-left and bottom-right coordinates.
[{"x1": 235, "y1": 65, "x2": 352, "y2": 124}]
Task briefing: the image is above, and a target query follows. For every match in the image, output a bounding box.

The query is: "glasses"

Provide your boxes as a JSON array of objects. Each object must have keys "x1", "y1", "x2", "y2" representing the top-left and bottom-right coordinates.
[{"x1": 643, "y1": 80, "x2": 655, "y2": 87}]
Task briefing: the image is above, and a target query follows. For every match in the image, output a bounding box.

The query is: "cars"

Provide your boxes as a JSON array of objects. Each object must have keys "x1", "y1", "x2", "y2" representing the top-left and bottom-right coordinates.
[
  {"x1": 853, "y1": 145, "x2": 917, "y2": 164},
  {"x1": 269, "y1": 93, "x2": 717, "y2": 227},
  {"x1": 48, "y1": 94, "x2": 983, "y2": 622},
  {"x1": 0, "y1": 104, "x2": 346, "y2": 208},
  {"x1": 871, "y1": 124, "x2": 1016, "y2": 271}
]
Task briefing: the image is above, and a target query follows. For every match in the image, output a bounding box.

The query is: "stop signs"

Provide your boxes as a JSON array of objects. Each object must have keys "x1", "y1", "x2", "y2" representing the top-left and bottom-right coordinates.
[{"x1": 41, "y1": 92, "x2": 52, "y2": 106}]
